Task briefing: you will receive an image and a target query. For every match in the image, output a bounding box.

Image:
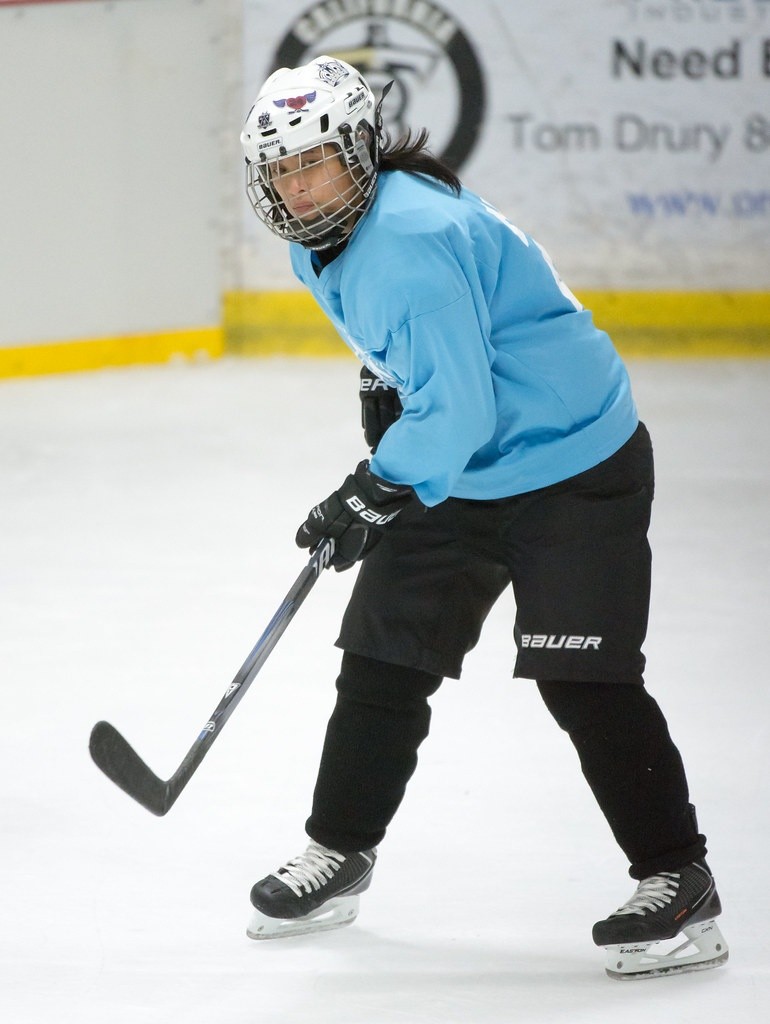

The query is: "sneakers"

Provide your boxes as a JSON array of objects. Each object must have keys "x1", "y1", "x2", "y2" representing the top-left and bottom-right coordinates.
[
  {"x1": 592, "y1": 854, "x2": 731, "y2": 979},
  {"x1": 243, "y1": 841, "x2": 374, "y2": 940}
]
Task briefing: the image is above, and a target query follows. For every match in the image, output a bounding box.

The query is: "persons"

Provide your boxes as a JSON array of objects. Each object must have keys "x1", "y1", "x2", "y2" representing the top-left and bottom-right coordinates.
[{"x1": 237, "y1": 54, "x2": 727, "y2": 949}]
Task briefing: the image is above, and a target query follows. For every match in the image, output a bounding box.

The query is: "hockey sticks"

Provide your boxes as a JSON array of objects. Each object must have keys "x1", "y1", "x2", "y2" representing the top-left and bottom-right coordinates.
[{"x1": 89, "y1": 536, "x2": 336, "y2": 819}]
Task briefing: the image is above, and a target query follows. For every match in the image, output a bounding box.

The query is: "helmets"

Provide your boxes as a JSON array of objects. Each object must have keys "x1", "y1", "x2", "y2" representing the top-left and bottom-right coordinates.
[{"x1": 240, "y1": 55, "x2": 375, "y2": 250}]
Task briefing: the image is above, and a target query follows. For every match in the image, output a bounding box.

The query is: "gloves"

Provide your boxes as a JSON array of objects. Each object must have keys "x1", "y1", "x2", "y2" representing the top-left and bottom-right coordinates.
[
  {"x1": 294, "y1": 457, "x2": 416, "y2": 572},
  {"x1": 358, "y1": 366, "x2": 406, "y2": 453}
]
